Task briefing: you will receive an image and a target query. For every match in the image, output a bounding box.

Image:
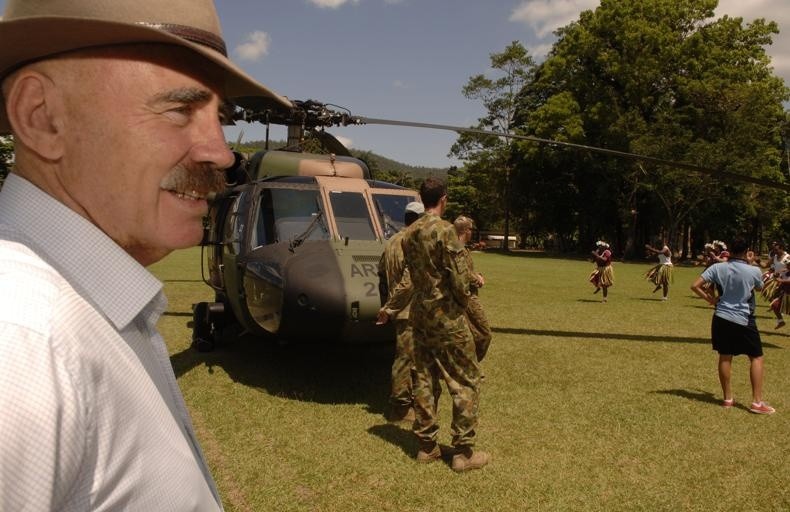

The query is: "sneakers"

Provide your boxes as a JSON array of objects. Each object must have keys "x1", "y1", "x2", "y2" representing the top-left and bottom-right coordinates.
[
  {"x1": 750, "y1": 401, "x2": 776, "y2": 414},
  {"x1": 720, "y1": 398, "x2": 737, "y2": 407},
  {"x1": 774, "y1": 320, "x2": 786, "y2": 330},
  {"x1": 416, "y1": 443, "x2": 454, "y2": 463},
  {"x1": 451, "y1": 452, "x2": 488, "y2": 473}
]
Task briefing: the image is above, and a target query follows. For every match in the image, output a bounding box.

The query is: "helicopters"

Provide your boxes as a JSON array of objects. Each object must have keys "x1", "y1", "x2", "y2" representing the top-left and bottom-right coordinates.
[{"x1": 192, "y1": 96, "x2": 789, "y2": 349}]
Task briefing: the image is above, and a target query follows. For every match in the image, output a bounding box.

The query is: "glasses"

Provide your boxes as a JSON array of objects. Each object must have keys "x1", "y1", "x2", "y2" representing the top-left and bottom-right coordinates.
[{"x1": 467, "y1": 225, "x2": 475, "y2": 233}]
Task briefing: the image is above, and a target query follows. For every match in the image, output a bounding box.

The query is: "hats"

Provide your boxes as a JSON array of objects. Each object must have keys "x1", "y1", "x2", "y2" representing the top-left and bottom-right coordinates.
[
  {"x1": 596, "y1": 240, "x2": 610, "y2": 249},
  {"x1": 703, "y1": 242, "x2": 713, "y2": 249},
  {"x1": 0, "y1": 1, "x2": 295, "y2": 111},
  {"x1": 405, "y1": 201, "x2": 425, "y2": 215},
  {"x1": 713, "y1": 240, "x2": 727, "y2": 251}
]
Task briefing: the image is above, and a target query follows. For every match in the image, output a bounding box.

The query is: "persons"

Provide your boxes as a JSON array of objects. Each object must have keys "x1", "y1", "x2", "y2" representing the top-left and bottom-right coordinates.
[
  {"x1": 588, "y1": 239, "x2": 614, "y2": 304},
  {"x1": 694, "y1": 239, "x2": 790, "y2": 330},
  {"x1": 645, "y1": 240, "x2": 674, "y2": 301},
  {"x1": 400, "y1": 177, "x2": 490, "y2": 471},
  {"x1": 0, "y1": 1, "x2": 295, "y2": 511},
  {"x1": 690, "y1": 235, "x2": 776, "y2": 414},
  {"x1": 451, "y1": 213, "x2": 492, "y2": 381},
  {"x1": 374, "y1": 200, "x2": 444, "y2": 423}
]
